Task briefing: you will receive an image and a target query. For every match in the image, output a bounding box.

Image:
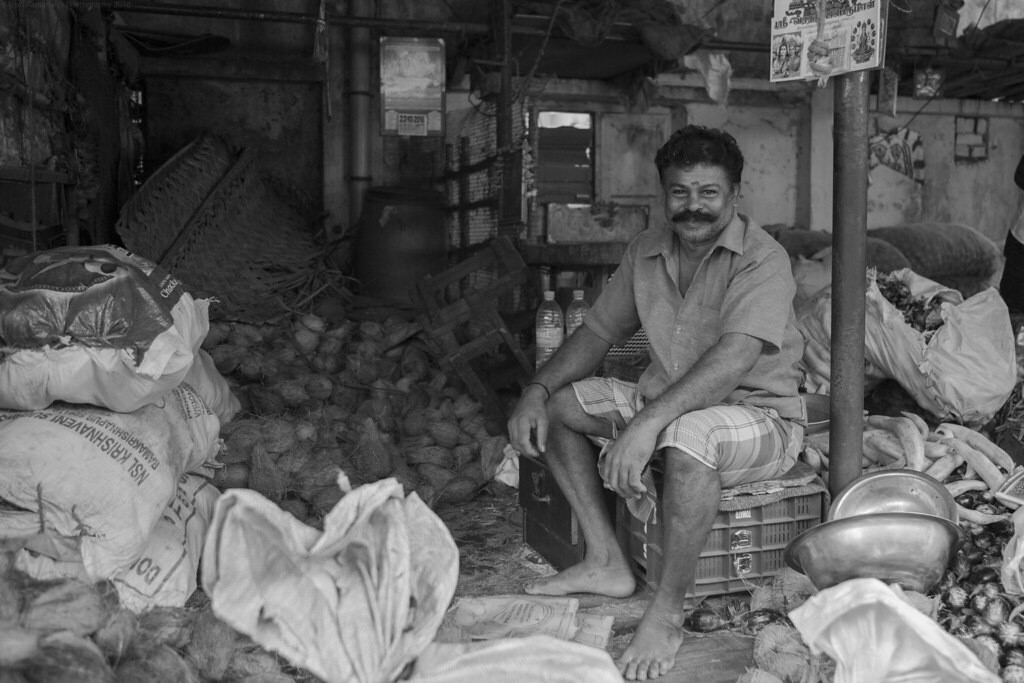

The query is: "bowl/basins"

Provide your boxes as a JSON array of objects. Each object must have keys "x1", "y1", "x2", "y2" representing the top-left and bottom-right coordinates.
[
  {"x1": 782, "y1": 512, "x2": 967, "y2": 596},
  {"x1": 802, "y1": 393, "x2": 831, "y2": 434},
  {"x1": 828, "y1": 470, "x2": 960, "y2": 528}
]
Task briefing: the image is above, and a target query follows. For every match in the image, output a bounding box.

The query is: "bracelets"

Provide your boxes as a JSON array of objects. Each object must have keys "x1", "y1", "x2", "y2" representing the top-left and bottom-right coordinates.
[{"x1": 528, "y1": 383, "x2": 550, "y2": 397}]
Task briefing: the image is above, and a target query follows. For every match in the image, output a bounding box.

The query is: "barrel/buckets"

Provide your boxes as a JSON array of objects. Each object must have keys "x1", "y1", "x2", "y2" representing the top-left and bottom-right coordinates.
[{"x1": 357, "y1": 188, "x2": 449, "y2": 300}]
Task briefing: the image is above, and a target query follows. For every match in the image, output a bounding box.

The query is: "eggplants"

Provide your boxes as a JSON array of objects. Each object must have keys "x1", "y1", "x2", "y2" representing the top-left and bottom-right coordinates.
[
  {"x1": 688, "y1": 600, "x2": 794, "y2": 639},
  {"x1": 930, "y1": 462, "x2": 1024, "y2": 683},
  {"x1": 879, "y1": 277, "x2": 952, "y2": 345}
]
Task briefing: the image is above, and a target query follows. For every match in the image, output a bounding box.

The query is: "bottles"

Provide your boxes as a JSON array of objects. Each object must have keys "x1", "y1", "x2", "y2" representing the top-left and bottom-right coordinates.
[
  {"x1": 537, "y1": 265, "x2": 588, "y2": 290},
  {"x1": 534, "y1": 292, "x2": 564, "y2": 373},
  {"x1": 565, "y1": 291, "x2": 591, "y2": 339}
]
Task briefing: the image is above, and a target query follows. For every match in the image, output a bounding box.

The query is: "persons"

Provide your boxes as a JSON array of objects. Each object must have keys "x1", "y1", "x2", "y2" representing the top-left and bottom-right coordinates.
[
  {"x1": 999, "y1": 154, "x2": 1023, "y2": 312},
  {"x1": 507, "y1": 125, "x2": 807, "y2": 680}
]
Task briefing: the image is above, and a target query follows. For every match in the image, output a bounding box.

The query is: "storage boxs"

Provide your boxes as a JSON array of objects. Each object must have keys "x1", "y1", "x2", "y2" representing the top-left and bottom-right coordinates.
[
  {"x1": 616, "y1": 476, "x2": 824, "y2": 603},
  {"x1": 519, "y1": 453, "x2": 620, "y2": 576}
]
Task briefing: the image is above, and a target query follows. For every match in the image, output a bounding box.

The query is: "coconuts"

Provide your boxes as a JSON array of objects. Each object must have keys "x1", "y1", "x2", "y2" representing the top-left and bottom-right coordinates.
[
  {"x1": 736, "y1": 566, "x2": 826, "y2": 683},
  {"x1": 1, "y1": 543, "x2": 305, "y2": 683},
  {"x1": 193, "y1": 313, "x2": 503, "y2": 527}
]
